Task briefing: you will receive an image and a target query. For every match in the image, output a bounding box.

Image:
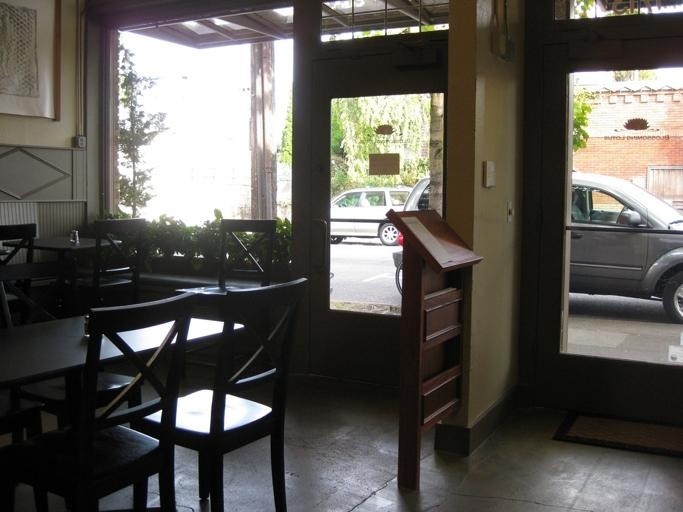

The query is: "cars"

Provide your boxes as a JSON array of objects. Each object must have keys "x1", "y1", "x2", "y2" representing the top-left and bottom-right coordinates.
[
  {"x1": 393, "y1": 167, "x2": 683, "y2": 329},
  {"x1": 329, "y1": 186, "x2": 418, "y2": 247}
]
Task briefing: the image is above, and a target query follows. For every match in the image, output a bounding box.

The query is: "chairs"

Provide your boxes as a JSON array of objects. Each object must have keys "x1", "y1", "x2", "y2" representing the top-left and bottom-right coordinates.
[
  {"x1": 2, "y1": 288, "x2": 199, "y2": 510},
  {"x1": 2, "y1": 221, "x2": 39, "y2": 324},
  {"x1": 50, "y1": 217, "x2": 148, "y2": 319},
  {"x1": 171, "y1": 217, "x2": 275, "y2": 379},
  {"x1": 132, "y1": 277, "x2": 306, "y2": 510}
]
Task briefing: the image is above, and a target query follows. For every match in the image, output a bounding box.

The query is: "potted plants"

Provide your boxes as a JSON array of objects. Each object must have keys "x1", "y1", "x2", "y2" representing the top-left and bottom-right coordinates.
[{"x1": 96, "y1": 212, "x2": 292, "y2": 279}]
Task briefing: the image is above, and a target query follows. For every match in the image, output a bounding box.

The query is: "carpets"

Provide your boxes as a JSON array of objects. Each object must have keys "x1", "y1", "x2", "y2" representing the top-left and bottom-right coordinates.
[{"x1": 547, "y1": 405, "x2": 683, "y2": 461}]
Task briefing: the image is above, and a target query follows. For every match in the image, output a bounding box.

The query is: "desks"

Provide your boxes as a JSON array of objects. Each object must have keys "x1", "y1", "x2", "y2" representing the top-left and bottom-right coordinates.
[
  {"x1": 0, "y1": 315, "x2": 246, "y2": 512},
  {"x1": 2, "y1": 234, "x2": 125, "y2": 317}
]
